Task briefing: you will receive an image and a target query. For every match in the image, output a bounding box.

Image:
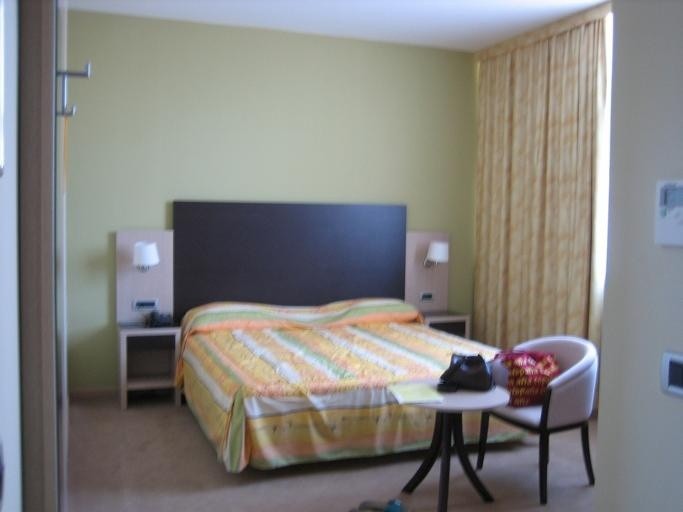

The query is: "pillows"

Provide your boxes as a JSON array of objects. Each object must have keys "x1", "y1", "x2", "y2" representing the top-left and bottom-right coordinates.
[{"x1": 494, "y1": 352, "x2": 559, "y2": 407}]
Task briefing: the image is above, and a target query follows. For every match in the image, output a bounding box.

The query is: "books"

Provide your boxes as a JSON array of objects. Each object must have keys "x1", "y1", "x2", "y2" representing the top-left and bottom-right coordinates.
[
  {"x1": 119, "y1": 319, "x2": 146, "y2": 329},
  {"x1": 387, "y1": 383, "x2": 445, "y2": 406}
]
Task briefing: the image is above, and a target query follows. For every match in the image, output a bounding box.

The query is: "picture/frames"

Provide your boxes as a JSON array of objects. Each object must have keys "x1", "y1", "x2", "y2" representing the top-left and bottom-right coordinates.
[{"x1": 655, "y1": 180, "x2": 683, "y2": 245}]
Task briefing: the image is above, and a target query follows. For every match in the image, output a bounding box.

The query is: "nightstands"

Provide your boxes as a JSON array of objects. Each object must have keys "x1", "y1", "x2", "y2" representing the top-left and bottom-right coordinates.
[
  {"x1": 424, "y1": 312, "x2": 471, "y2": 339},
  {"x1": 118, "y1": 326, "x2": 182, "y2": 410}
]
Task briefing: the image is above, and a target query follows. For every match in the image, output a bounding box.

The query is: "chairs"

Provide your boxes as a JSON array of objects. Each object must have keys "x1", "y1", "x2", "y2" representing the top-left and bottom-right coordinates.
[{"x1": 477, "y1": 335, "x2": 598, "y2": 504}]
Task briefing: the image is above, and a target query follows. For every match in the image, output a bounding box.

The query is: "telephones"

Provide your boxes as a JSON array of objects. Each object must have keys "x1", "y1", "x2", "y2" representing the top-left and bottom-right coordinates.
[{"x1": 145, "y1": 311, "x2": 171, "y2": 325}]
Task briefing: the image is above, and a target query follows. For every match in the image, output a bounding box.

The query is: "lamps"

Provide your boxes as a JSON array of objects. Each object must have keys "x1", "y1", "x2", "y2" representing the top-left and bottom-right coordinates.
[
  {"x1": 424, "y1": 241, "x2": 449, "y2": 268},
  {"x1": 133, "y1": 241, "x2": 160, "y2": 273}
]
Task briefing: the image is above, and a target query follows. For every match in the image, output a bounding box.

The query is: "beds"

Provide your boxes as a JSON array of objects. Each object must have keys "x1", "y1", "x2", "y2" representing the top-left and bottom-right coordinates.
[{"x1": 173, "y1": 202, "x2": 528, "y2": 474}]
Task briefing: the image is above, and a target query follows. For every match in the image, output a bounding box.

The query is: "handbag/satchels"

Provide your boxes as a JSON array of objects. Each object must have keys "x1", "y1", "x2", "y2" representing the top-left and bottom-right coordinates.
[
  {"x1": 435, "y1": 351, "x2": 495, "y2": 393},
  {"x1": 489, "y1": 349, "x2": 560, "y2": 408}
]
